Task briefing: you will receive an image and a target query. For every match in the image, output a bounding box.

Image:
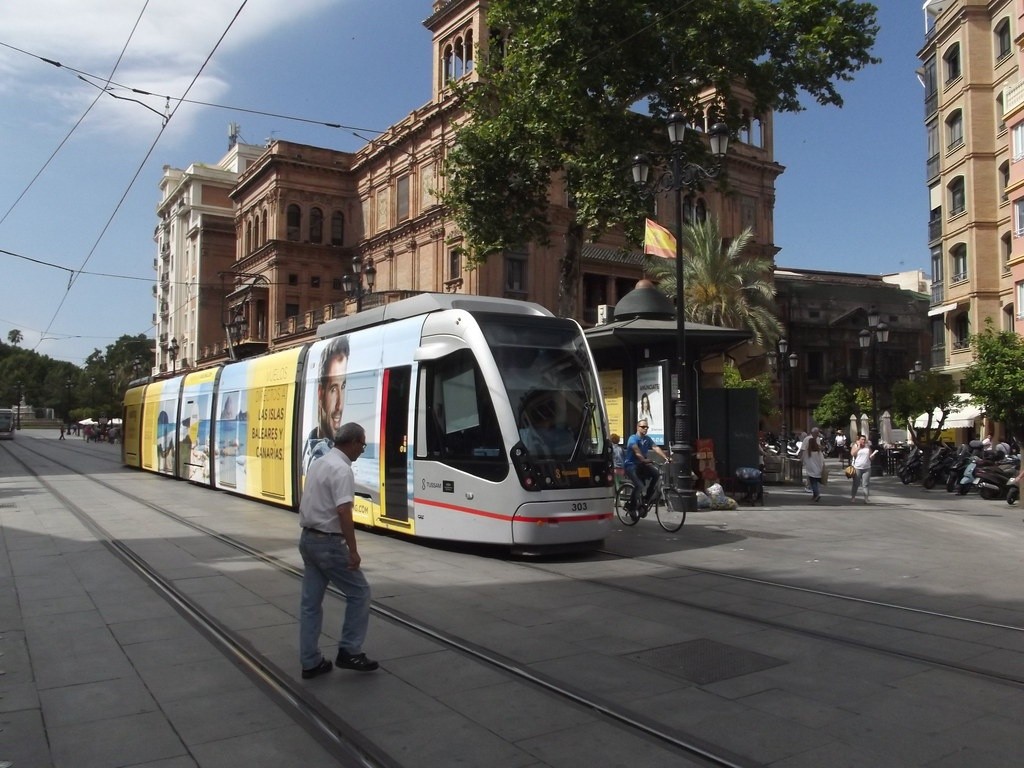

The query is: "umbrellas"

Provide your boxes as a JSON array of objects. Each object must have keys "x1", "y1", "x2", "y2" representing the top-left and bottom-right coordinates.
[{"x1": 79, "y1": 418, "x2": 123, "y2": 425}]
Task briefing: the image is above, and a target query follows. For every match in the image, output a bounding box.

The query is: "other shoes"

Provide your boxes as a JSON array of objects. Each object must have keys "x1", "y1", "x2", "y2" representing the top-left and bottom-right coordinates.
[
  {"x1": 805, "y1": 488, "x2": 813, "y2": 492},
  {"x1": 865, "y1": 500, "x2": 870, "y2": 503},
  {"x1": 630, "y1": 499, "x2": 637, "y2": 507},
  {"x1": 815, "y1": 496, "x2": 821, "y2": 502},
  {"x1": 851, "y1": 498, "x2": 855, "y2": 502}
]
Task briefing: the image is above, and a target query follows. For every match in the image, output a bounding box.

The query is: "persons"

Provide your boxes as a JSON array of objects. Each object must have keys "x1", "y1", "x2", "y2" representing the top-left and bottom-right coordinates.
[
  {"x1": 72, "y1": 424, "x2": 78, "y2": 434},
  {"x1": 301, "y1": 335, "x2": 350, "y2": 476},
  {"x1": 759, "y1": 444, "x2": 769, "y2": 494},
  {"x1": 788, "y1": 427, "x2": 846, "y2": 493},
  {"x1": 803, "y1": 438, "x2": 825, "y2": 503},
  {"x1": 59, "y1": 425, "x2": 66, "y2": 440},
  {"x1": 157, "y1": 418, "x2": 192, "y2": 479},
  {"x1": 975, "y1": 433, "x2": 1020, "y2": 457},
  {"x1": 610, "y1": 434, "x2": 626, "y2": 507},
  {"x1": 624, "y1": 420, "x2": 672, "y2": 521},
  {"x1": 298, "y1": 422, "x2": 379, "y2": 679},
  {"x1": 639, "y1": 393, "x2": 654, "y2": 423},
  {"x1": 1014, "y1": 470, "x2": 1024, "y2": 522},
  {"x1": 849, "y1": 435, "x2": 875, "y2": 503},
  {"x1": 84, "y1": 425, "x2": 118, "y2": 444}
]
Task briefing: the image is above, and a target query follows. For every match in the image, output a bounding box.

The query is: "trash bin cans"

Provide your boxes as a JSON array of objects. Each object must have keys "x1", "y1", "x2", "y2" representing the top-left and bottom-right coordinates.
[{"x1": 871, "y1": 445, "x2": 885, "y2": 476}]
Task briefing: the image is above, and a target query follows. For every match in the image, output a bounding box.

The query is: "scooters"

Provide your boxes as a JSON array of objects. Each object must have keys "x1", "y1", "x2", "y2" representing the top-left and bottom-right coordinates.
[
  {"x1": 741, "y1": 464, "x2": 768, "y2": 507},
  {"x1": 764, "y1": 439, "x2": 850, "y2": 459},
  {"x1": 893, "y1": 441, "x2": 1024, "y2": 504}
]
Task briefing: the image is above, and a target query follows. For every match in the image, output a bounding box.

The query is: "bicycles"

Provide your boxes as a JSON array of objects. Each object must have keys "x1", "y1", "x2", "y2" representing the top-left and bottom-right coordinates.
[{"x1": 616, "y1": 458, "x2": 685, "y2": 533}]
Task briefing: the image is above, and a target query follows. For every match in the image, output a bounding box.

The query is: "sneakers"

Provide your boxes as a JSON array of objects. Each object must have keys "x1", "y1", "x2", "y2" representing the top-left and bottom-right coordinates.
[
  {"x1": 335, "y1": 652, "x2": 379, "y2": 670},
  {"x1": 302, "y1": 657, "x2": 332, "y2": 677}
]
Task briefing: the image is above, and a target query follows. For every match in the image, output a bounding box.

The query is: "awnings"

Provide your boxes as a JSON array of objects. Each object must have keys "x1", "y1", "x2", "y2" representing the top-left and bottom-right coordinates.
[{"x1": 914, "y1": 393, "x2": 989, "y2": 429}]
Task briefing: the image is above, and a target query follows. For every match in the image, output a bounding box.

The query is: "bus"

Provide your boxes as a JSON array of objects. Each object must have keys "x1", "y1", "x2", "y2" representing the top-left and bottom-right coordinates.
[{"x1": 120, "y1": 293, "x2": 615, "y2": 557}]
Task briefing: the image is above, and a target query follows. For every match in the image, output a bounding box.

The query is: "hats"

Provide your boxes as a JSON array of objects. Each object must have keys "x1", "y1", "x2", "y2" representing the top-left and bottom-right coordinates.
[{"x1": 836, "y1": 430, "x2": 844, "y2": 434}]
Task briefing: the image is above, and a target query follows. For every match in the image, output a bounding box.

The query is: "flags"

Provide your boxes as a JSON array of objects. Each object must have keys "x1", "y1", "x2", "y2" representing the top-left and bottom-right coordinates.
[{"x1": 644, "y1": 217, "x2": 676, "y2": 258}]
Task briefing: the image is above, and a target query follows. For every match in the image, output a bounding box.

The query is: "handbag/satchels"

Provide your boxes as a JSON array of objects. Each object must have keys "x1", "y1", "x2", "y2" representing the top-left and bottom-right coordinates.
[
  {"x1": 819, "y1": 462, "x2": 829, "y2": 485},
  {"x1": 846, "y1": 466, "x2": 856, "y2": 478}
]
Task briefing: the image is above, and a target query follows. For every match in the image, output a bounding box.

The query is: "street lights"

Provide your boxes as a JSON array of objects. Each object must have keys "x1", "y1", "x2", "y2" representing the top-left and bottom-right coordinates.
[
  {"x1": 168, "y1": 337, "x2": 179, "y2": 378},
  {"x1": 629, "y1": 107, "x2": 728, "y2": 512},
  {"x1": 858, "y1": 303, "x2": 889, "y2": 477},
  {"x1": 341, "y1": 254, "x2": 377, "y2": 313},
  {"x1": 65, "y1": 376, "x2": 74, "y2": 436},
  {"x1": 766, "y1": 338, "x2": 799, "y2": 487},
  {"x1": 89, "y1": 373, "x2": 96, "y2": 411},
  {"x1": 229, "y1": 310, "x2": 248, "y2": 355},
  {"x1": 132, "y1": 355, "x2": 141, "y2": 380},
  {"x1": 108, "y1": 367, "x2": 116, "y2": 418},
  {"x1": 13, "y1": 379, "x2": 25, "y2": 431}
]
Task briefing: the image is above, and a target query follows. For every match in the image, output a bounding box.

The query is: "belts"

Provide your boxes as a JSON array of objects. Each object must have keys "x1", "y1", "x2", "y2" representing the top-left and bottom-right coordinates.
[{"x1": 302, "y1": 526, "x2": 344, "y2": 536}]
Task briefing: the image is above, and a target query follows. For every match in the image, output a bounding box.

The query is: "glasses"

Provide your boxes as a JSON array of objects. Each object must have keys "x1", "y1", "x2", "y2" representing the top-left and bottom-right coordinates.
[
  {"x1": 359, "y1": 441, "x2": 367, "y2": 449},
  {"x1": 639, "y1": 426, "x2": 649, "y2": 429}
]
